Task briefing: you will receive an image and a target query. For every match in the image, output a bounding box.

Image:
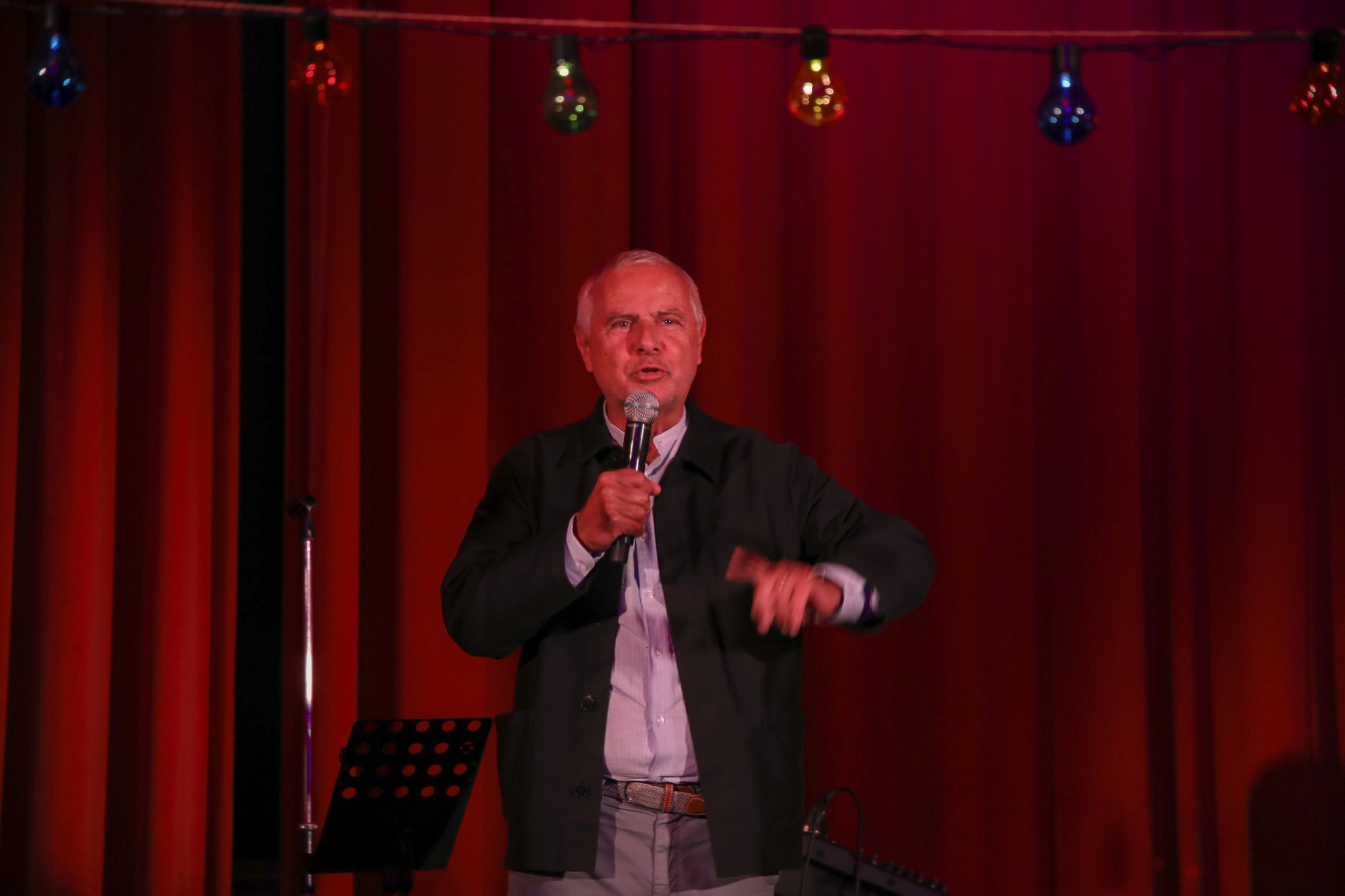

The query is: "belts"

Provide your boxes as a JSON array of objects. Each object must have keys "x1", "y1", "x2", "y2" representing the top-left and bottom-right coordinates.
[{"x1": 602, "y1": 777, "x2": 705, "y2": 817}]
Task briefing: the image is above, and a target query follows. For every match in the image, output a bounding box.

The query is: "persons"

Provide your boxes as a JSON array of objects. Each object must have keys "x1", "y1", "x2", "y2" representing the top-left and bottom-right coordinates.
[{"x1": 441, "y1": 251, "x2": 935, "y2": 896}]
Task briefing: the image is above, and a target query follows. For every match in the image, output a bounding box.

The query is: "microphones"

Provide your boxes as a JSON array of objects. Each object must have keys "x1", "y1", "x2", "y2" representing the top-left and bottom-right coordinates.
[{"x1": 608, "y1": 389, "x2": 659, "y2": 565}]
